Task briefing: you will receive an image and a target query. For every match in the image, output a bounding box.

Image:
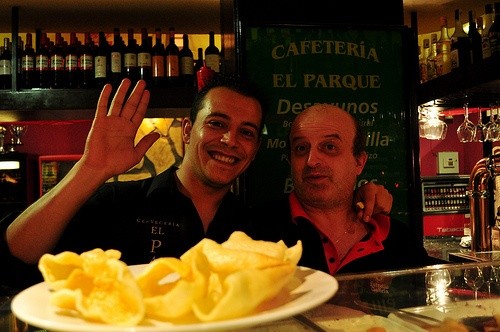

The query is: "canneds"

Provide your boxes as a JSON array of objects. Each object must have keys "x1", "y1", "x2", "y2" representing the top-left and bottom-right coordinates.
[{"x1": 42, "y1": 161, "x2": 59, "y2": 194}]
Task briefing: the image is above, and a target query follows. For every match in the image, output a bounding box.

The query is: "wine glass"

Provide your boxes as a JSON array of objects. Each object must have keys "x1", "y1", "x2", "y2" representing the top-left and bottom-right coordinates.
[
  {"x1": 418, "y1": 104, "x2": 500, "y2": 142},
  {"x1": 426, "y1": 265, "x2": 500, "y2": 306}
]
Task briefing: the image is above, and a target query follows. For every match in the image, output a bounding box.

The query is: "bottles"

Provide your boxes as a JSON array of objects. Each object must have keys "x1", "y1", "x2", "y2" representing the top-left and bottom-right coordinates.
[
  {"x1": 466, "y1": 10, "x2": 482, "y2": 65},
  {"x1": 428, "y1": 33, "x2": 438, "y2": 82},
  {"x1": 450, "y1": 8, "x2": 470, "y2": 71},
  {"x1": 0, "y1": 29, "x2": 220, "y2": 92},
  {"x1": 419, "y1": 45, "x2": 422, "y2": 84},
  {"x1": 488, "y1": 0, "x2": 500, "y2": 56},
  {"x1": 482, "y1": 3, "x2": 494, "y2": 61},
  {"x1": 476, "y1": 16, "x2": 484, "y2": 35},
  {"x1": 437, "y1": 16, "x2": 451, "y2": 77},
  {"x1": 419, "y1": 38, "x2": 430, "y2": 84}
]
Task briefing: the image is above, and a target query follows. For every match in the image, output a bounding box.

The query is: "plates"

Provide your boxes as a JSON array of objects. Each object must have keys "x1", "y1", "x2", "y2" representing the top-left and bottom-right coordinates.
[{"x1": 10, "y1": 263, "x2": 338, "y2": 331}]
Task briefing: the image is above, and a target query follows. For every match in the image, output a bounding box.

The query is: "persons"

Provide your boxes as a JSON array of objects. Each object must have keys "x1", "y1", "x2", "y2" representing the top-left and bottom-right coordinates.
[
  {"x1": 261, "y1": 104, "x2": 429, "y2": 295},
  {"x1": 0, "y1": 72, "x2": 393, "y2": 297}
]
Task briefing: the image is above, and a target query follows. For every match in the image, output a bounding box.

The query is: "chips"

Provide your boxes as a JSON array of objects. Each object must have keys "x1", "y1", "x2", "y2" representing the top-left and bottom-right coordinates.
[{"x1": 38, "y1": 231, "x2": 302, "y2": 324}]
